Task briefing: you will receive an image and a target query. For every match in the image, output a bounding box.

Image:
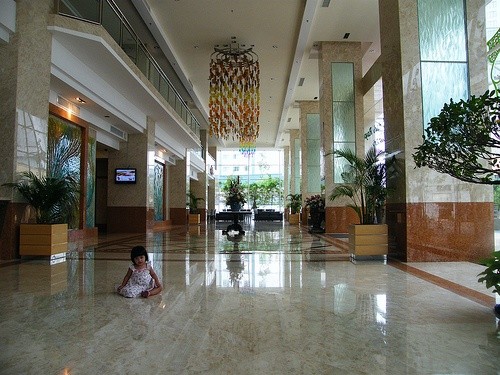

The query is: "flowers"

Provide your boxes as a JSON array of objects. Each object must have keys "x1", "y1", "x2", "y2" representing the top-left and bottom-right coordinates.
[{"x1": 223, "y1": 181, "x2": 247, "y2": 206}]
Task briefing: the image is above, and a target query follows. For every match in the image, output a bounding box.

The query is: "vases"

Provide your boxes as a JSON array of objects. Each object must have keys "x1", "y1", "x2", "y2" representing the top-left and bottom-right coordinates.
[{"x1": 230, "y1": 202, "x2": 242, "y2": 210}]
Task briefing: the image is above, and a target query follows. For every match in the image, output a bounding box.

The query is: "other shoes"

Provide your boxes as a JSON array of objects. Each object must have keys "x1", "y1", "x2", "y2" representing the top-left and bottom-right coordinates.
[{"x1": 141, "y1": 290, "x2": 150, "y2": 298}]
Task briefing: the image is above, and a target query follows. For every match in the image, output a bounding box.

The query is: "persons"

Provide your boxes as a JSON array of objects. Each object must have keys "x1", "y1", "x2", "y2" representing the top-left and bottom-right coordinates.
[{"x1": 115, "y1": 245, "x2": 162, "y2": 298}]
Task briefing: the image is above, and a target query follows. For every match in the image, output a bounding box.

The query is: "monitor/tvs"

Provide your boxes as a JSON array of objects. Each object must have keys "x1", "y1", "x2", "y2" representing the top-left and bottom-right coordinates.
[{"x1": 114, "y1": 168, "x2": 136, "y2": 184}]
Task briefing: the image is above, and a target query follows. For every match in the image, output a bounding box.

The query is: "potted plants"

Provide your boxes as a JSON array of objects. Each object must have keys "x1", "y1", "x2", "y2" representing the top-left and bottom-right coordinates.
[
  {"x1": 185, "y1": 190, "x2": 205, "y2": 223},
  {"x1": 286, "y1": 193, "x2": 303, "y2": 226},
  {"x1": 302, "y1": 194, "x2": 325, "y2": 233},
  {"x1": 323, "y1": 147, "x2": 396, "y2": 261},
  {"x1": 0, "y1": 171, "x2": 86, "y2": 260}
]
